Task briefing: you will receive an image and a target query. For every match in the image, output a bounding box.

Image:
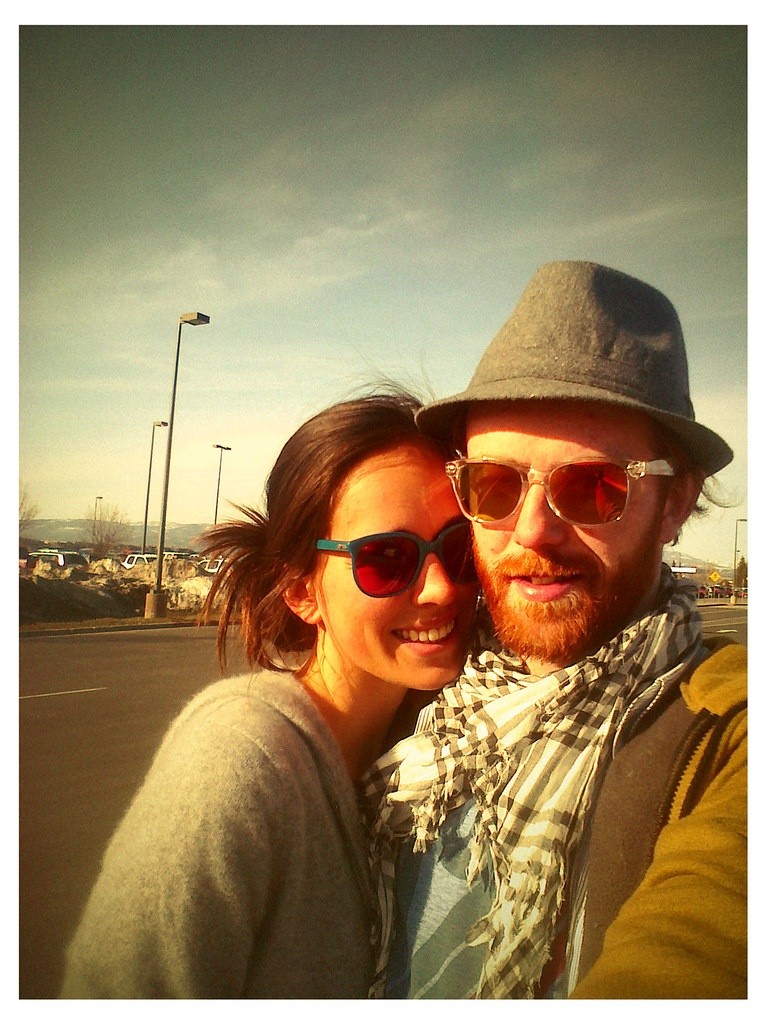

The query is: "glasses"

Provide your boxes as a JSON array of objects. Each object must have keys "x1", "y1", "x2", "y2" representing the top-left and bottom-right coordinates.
[
  {"x1": 448, "y1": 460, "x2": 686, "y2": 528},
  {"x1": 317, "y1": 514, "x2": 479, "y2": 599}
]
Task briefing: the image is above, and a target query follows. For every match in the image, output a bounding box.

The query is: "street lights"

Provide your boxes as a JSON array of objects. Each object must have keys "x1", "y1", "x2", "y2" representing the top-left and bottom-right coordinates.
[
  {"x1": 143, "y1": 311, "x2": 210, "y2": 617},
  {"x1": 141, "y1": 420, "x2": 167, "y2": 554},
  {"x1": 213, "y1": 442, "x2": 232, "y2": 525},
  {"x1": 730, "y1": 518, "x2": 747, "y2": 603},
  {"x1": 92, "y1": 497, "x2": 103, "y2": 541}
]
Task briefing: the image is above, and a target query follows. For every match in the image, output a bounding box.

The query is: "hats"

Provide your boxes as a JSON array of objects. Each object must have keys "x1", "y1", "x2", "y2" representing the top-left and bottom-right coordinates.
[{"x1": 415, "y1": 258, "x2": 735, "y2": 474}]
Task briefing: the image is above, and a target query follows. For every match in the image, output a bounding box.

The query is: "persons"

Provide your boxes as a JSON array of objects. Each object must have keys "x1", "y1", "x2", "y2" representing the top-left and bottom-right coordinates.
[
  {"x1": 64, "y1": 398, "x2": 476, "y2": 1000},
  {"x1": 367, "y1": 261, "x2": 747, "y2": 998}
]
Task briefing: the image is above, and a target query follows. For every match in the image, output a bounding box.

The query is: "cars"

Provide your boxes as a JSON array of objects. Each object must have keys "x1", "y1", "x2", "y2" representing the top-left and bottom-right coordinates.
[
  {"x1": 694, "y1": 584, "x2": 747, "y2": 600},
  {"x1": 120, "y1": 550, "x2": 223, "y2": 579}
]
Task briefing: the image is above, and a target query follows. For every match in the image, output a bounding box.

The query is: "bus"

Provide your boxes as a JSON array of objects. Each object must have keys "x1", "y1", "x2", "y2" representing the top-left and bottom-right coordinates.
[{"x1": 26, "y1": 547, "x2": 89, "y2": 572}]
